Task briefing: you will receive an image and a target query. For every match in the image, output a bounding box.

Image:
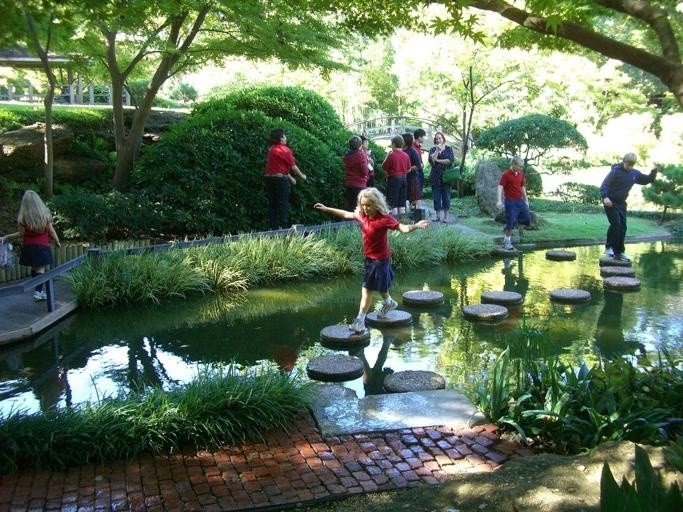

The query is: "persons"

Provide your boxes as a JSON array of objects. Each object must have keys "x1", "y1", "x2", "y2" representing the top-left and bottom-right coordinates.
[
  {"x1": 410, "y1": 129, "x2": 425, "y2": 170},
  {"x1": 600, "y1": 152, "x2": 661, "y2": 260},
  {"x1": 381, "y1": 135, "x2": 410, "y2": 218},
  {"x1": 400, "y1": 132, "x2": 422, "y2": 212},
  {"x1": 428, "y1": 131, "x2": 454, "y2": 224},
  {"x1": 359, "y1": 135, "x2": 375, "y2": 190},
  {"x1": 495, "y1": 157, "x2": 531, "y2": 252},
  {"x1": 261, "y1": 128, "x2": 309, "y2": 230},
  {"x1": 341, "y1": 136, "x2": 369, "y2": 215},
  {"x1": 0, "y1": 188, "x2": 61, "y2": 301},
  {"x1": 310, "y1": 186, "x2": 427, "y2": 333}
]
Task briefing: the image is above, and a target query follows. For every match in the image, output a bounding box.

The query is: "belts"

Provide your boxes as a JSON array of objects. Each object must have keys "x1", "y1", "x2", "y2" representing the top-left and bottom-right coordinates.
[{"x1": 264, "y1": 172, "x2": 288, "y2": 178}]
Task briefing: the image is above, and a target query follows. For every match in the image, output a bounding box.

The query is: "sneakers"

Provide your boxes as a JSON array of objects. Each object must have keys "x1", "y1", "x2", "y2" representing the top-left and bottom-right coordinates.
[
  {"x1": 346, "y1": 317, "x2": 366, "y2": 332},
  {"x1": 501, "y1": 240, "x2": 515, "y2": 251},
  {"x1": 32, "y1": 290, "x2": 47, "y2": 301},
  {"x1": 605, "y1": 248, "x2": 632, "y2": 263},
  {"x1": 377, "y1": 300, "x2": 399, "y2": 318},
  {"x1": 432, "y1": 216, "x2": 450, "y2": 224}
]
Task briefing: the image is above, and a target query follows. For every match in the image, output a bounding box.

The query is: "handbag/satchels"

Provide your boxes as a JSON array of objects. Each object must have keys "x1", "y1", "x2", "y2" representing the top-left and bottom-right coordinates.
[{"x1": 441, "y1": 161, "x2": 462, "y2": 182}]
